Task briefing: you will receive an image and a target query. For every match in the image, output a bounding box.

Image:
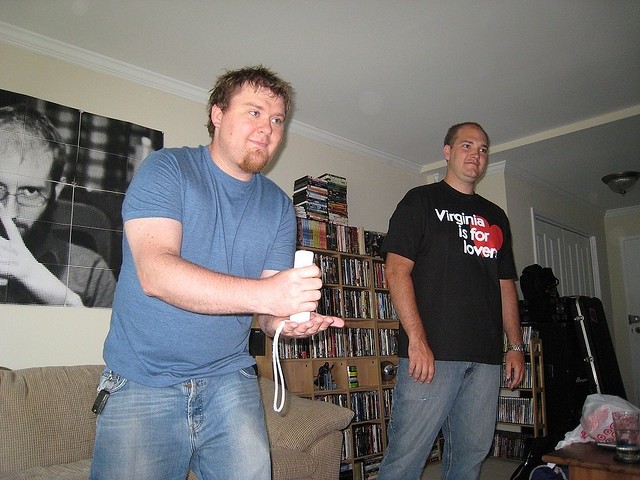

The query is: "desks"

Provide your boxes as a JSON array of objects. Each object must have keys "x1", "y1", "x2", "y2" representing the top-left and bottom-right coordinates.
[{"x1": 540, "y1": 438, "x2": 640, "y2": 480}]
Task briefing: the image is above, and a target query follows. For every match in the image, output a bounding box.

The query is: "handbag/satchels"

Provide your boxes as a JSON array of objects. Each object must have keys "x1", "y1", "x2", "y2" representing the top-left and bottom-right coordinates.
[{"x1": 508, "y1": 449, "x2": 570, "y2": 480}]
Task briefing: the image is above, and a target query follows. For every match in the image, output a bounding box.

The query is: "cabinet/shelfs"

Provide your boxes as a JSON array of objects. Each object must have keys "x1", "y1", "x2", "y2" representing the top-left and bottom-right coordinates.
[
  {"x1": 252, "y1": 244, "x2": 441, "y2": 480},
  {"x1": 489, "y1": 340, "x2": 548, "y2": 462}
]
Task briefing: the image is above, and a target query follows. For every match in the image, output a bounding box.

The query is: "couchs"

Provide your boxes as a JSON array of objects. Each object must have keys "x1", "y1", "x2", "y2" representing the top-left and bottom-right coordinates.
[{"x1": 1, "y1": 361, "x2": 358, "y2": 480}]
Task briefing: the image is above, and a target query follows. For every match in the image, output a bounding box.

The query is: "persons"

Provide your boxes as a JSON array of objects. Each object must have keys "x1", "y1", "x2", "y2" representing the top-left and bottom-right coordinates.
[
  {"x1": 87, "y1": 67, "x2": 345, "y2": 479},
  {"x1": 314, "y1": 362, "x2": 337, "y2": 391},
  {"x1": 0, "y1": 103, "x2": 116, "y2": 307},
  {"x1": 375, "y1": 121, "x2": 526, "y2": 480}
]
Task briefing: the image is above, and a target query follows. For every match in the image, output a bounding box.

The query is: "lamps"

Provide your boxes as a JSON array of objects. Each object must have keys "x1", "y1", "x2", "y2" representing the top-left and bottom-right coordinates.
[{"x1": 600, "y1": 170, "x2": 640, "y2": 195}]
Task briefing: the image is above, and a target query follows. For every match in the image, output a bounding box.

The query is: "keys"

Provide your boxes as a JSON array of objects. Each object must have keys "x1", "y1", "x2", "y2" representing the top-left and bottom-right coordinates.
[{"x1": 91, "y1": 389, "x2": 109, "y2": 414}]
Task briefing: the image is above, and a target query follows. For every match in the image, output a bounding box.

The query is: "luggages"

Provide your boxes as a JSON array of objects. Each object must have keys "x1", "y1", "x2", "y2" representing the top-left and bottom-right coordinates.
[{"x1": 541, "y1": 295, "x2": 627, "y2": 423}]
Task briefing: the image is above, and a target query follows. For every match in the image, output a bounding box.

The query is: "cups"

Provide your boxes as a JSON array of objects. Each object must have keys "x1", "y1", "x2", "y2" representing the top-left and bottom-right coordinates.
[{"x1": 611, "y1": 411, "x2": 640, "y2": 462}]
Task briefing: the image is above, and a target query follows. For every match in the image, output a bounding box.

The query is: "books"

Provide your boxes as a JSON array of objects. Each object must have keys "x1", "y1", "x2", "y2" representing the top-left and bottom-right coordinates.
[
  {"x1": 500, "y1": 363, "x2": 540, "y2": 389},
  {"x1": 382, "y1": 389, "x2": 393, "y2": 418},
  {"x1": 375, "y1": 292, "x2": 399, "y2": 321},
  {"x1": 340, "y1": 429, "x2": 351, "y2": 462},
  {"x1": 293, "y1": 172, "x2": 349, "y2": 223},
  {"x1": 309, "y1": 254, "x2": 339, "y2": 284},
  {"x1": 278, "y1": 328, "x2": 345, "y2": 359},
  {"x1": 350, "y1": 391, "x2": 380, "y2": 422},
  {"x1": 346, "y1": 327, "x2": 375, "y2": 356},
  {"x1": 503, "y1": 326, "x2": 540, "y2": 355},
  {"x1": 313, "y1": 289, "x2": 342, "y2": 318},
  {"x1": 378, "y1": 328, "x2": 399, "y2": 356},
  {"x1": 499, "y1": 396, "x2": 542, "y2": 427},
  {"x1": 353, "y1": 424, "x2": 384, "y2": 458},
  {"x1": 298, "y1": 219, "x2": 359, "y2": 258},
  {"x1": 488, "y1": 433, "x2": 533, "y2": 459},
  {"x1": 365, "y1": 231, "x2": 387, "y2": 257},
  {"x1": 364, "y1": 456, "x2": 383, "y2": 480},
  {"x1": 343, "y1": 290, "x2": 371, "y2": 319},
  {"x1": 316, "y1": 393, "x2": 348, "y2": 409},
  {"x1": 374, "y1": 262, "x2": 388, "y2": 289},
  {"x1": 341, "y1": 257, "x2": 370, "y2": 287}
]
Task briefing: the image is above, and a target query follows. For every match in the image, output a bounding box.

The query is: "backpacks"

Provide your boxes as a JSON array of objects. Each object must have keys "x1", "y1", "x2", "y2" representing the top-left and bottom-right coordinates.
[{"x1": 520, "y1": 263, "x2": 559, "y2": 321}]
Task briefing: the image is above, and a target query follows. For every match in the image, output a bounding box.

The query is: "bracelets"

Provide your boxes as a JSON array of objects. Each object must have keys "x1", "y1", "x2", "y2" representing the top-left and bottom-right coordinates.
[{"x1": 507, "y1": 344, "x2": 525, "y2": 351}]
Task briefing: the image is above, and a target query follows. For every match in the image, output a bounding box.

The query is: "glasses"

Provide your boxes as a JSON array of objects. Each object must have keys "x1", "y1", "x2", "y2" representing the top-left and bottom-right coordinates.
[{"x1": 0, "y1": 180, "x2": 59, "y2": 208}]
